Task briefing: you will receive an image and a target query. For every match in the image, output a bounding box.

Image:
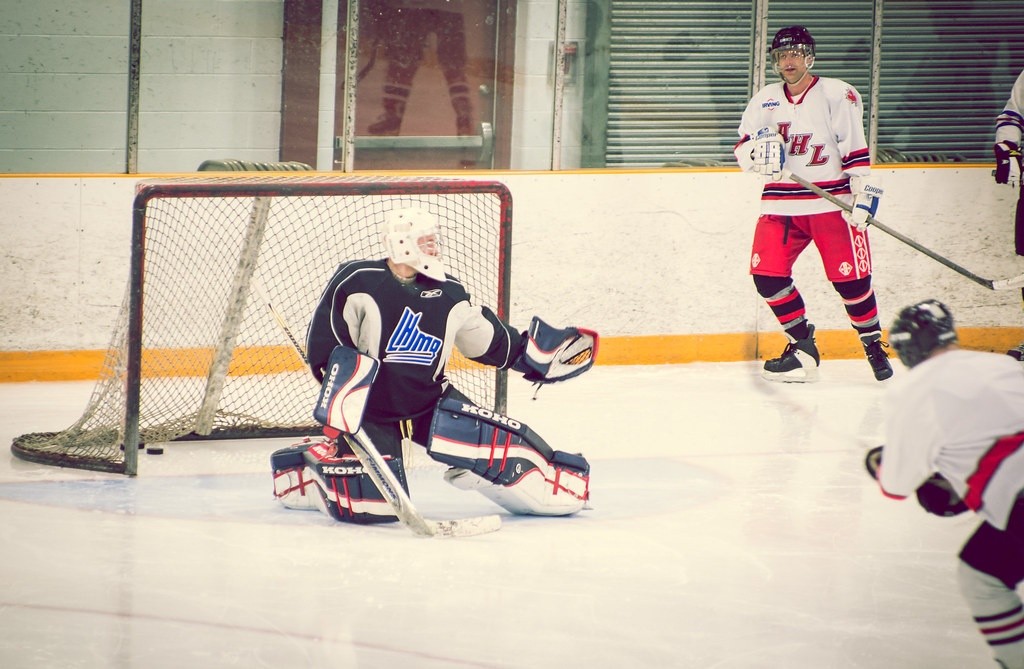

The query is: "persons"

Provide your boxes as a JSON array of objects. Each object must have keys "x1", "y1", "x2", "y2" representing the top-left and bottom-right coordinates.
[
  {"x1": 735, "y1": 26, "x2": 900, "y2": 385},
  {"x1": 368, "y1": 0, "x2": 474, "y2": 134},
  {"x1": 271, "y1": 207, "x2": 598, "y2": 518},
  {"x1": 867, "y1": 300, "x2": 1024, "y2": 669},
  {"x1": 994, "y1": 65, "x2": 1024, "y2": 257}
]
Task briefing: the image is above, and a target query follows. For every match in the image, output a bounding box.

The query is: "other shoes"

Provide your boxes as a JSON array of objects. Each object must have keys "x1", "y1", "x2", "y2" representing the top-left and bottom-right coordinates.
[{"x1": 1007, "y1": 342, "x2": 1024, "y2": 361}]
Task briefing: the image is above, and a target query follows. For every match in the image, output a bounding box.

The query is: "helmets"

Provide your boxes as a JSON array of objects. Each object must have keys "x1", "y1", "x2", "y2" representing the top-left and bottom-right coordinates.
[
  {"x1": 382, "y1": 208, "x2": 447, "y2": 283},
  {"x1": 890, "y1": 299, "x2": 958, "y2": 369},
  {"x1": 768, "y1": 25, "x2": 815, "y2": 76}
]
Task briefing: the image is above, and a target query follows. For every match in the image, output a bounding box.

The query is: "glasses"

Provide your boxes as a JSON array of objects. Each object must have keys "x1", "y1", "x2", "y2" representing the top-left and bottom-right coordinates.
[{"x1": 420, "y1": 240, "x2": 442, "y2": 250}]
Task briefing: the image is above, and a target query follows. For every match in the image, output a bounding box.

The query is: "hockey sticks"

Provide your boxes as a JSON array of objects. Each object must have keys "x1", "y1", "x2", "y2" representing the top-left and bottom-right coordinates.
[
  {"x1": 341, "y1": 17, "x2": 381, "y2": 90},
  {"x1": 749, "y1": 151, "x2": 1024, "y2": 292},
  {"x1": 248, "y1": 272, "x2": 504, "y2": 541}
]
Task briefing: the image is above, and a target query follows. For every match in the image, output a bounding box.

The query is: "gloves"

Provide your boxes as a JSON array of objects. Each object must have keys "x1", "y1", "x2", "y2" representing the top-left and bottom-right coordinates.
[
  {"x1": 752, "y1": 126, "x2": 785, "y2": 180},
  {"x1": 994, "y1": 140, "x2": 1024, "y2": 188},
  {"x1": 916, "y1": 473, "x2": 969, "y2": 517},
  {"x1": 842, "y1": 173, "x2": 893, "y2": 231}
]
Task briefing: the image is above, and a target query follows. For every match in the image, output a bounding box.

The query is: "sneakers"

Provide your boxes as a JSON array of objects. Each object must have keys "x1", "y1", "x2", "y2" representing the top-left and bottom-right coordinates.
[
  {"x1": 764, "y1": 324, "x2": 824, "y2": 383},
  {"x1": 860, "y1": 332, "x2": 892, "y2": 381}
]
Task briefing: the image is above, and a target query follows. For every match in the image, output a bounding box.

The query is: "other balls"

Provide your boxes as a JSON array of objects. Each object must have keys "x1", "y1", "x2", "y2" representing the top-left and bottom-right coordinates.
[
  {"x1": 138, "y1": 443, "x2": 144, "y2": 449},
  {"x1": 146, "y1": 447, "x2": 164, "y2": 454}
]
